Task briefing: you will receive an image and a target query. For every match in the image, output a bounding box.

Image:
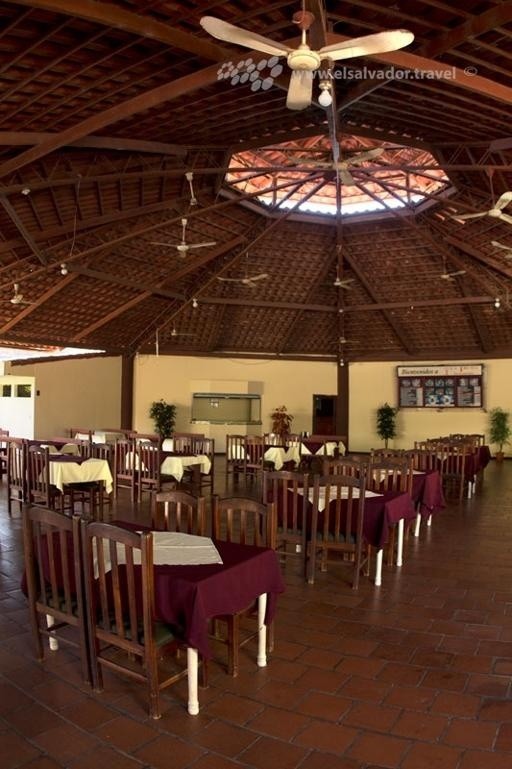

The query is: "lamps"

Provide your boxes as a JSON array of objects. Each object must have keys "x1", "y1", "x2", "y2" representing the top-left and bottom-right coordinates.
[{"x1": 316, "y1": 58, "x2": 334, "y2": 108}]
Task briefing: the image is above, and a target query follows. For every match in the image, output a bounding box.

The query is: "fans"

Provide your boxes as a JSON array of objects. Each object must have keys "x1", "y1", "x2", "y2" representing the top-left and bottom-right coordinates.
[{"x1": 200, "y1": 2, "x2": 416, "y2": 111}]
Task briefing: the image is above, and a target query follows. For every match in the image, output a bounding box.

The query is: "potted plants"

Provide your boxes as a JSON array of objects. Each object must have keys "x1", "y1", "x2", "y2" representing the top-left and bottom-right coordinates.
[{"x1": 487, "y1": 406, "x2": 511, "y2": 462}]
